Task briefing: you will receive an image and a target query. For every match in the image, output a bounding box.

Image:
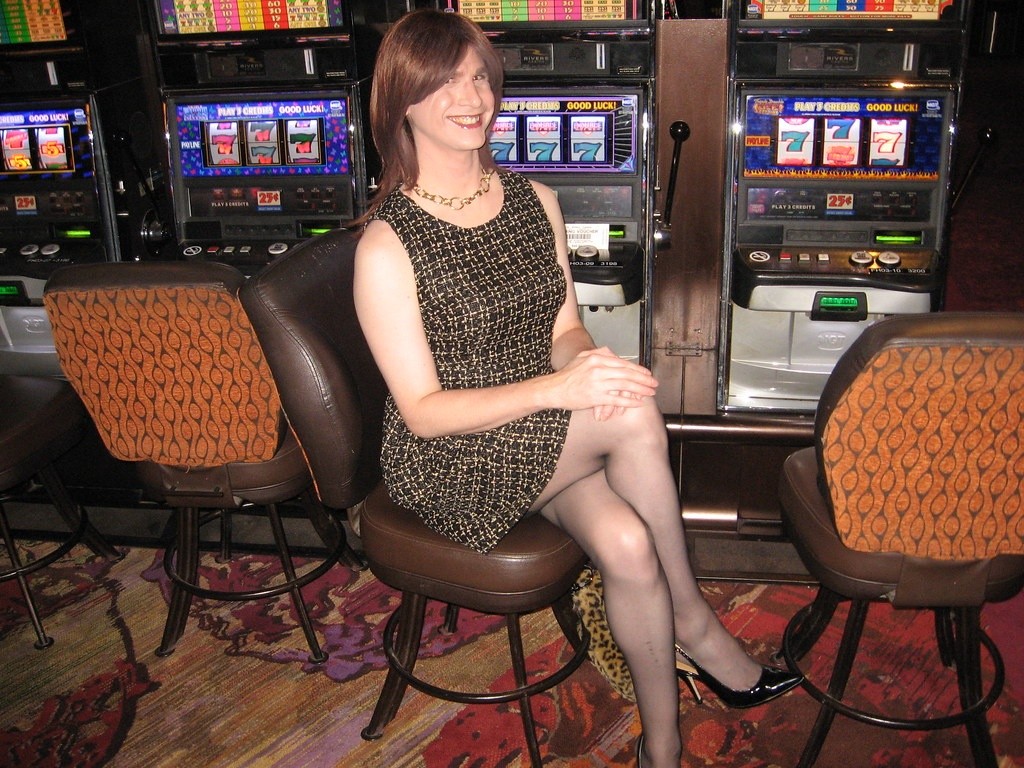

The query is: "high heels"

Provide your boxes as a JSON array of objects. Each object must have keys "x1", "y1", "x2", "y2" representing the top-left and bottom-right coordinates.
[{"x1": 674, "y1": 644, "x2": 805, "y2": 709}]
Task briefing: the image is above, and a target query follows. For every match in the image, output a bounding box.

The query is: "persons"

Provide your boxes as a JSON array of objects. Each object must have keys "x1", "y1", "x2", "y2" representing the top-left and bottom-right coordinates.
[{"x1": 351, "y1": 8, "x2": 808, "y2": 768}]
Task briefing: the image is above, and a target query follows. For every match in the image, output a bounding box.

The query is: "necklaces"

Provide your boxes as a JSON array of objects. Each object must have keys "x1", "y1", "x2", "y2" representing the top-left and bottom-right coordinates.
[{"x1": 412, "y1": 163, "x2": 492, "y2": 211}]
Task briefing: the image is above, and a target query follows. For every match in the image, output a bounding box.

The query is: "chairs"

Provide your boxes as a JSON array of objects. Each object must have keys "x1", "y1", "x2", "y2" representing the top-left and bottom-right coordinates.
[
  {"x1": 778, "y1": 312, "x2": 1024, "y2": 768},
  {"x1": 43, "y1": 261, "x2": 369, "y2": 665},
  {"x1": 0, "y1": 373, "x2": 125, "y2": 650},
  {"x1": 236, "y1": 226, "x2": 590, "y2": 768}
]
